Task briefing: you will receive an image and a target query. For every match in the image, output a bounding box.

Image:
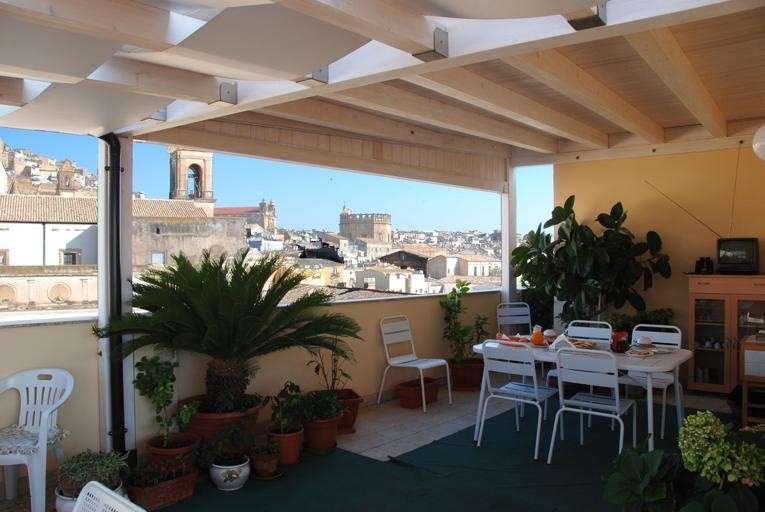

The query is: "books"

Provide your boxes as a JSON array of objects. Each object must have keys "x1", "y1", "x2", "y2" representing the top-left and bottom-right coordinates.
[{"x1": 756, "y1": 330, "x2": 765, "y2": 341}]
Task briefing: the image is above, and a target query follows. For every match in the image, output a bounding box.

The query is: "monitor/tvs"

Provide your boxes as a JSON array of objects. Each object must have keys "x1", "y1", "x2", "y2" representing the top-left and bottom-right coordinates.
[{"x1": 716, "y1": 238, "x2": 759, "y2": 275}]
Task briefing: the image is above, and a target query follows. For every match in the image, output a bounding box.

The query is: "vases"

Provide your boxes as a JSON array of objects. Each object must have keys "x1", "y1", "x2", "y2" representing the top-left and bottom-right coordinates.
[{"x1": 397, "y1": 376, "x2": 443, "y2": 409}]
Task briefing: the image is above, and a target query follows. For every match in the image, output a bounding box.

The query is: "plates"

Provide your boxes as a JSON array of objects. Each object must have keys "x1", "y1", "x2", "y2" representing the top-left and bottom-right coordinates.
[{"x1": 498, "y1": 324, "x2": 679, "y2": 361}]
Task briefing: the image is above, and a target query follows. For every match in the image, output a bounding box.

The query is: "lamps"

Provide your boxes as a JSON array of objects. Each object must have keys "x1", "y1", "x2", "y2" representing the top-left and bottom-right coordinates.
[{"x1": 752, "y1": 124, "x2": 765, "y2": 161}]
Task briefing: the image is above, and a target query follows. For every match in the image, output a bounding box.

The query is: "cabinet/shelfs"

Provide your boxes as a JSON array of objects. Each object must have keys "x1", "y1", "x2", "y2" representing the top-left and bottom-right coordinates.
[
  {"x1": 682, "y1": 273, "x2": 765, "y2": 396},
  {"x1": 735, "y1": 333, "x2": 765, "y2": 435}
]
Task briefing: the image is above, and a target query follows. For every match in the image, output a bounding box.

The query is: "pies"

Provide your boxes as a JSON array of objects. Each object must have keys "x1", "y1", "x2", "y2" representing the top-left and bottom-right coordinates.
[{"x1": 572, "y1": 341, "x2": 592, "y2": 348}]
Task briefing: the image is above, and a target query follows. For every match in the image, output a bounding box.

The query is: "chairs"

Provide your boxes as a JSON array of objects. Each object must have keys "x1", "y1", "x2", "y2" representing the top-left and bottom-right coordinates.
[
  {"x1": 474, "y1": 341, "x2": 567, "y2": 461},
  {"x1": 545, "y1": 347, "x2": 641, "y2": 466},
  {"x1": 493, "y1": 301, "x2": 557, "y2": 417},
  {"x1": 375, "y1": 313, "x2": 453, "y2": 413},
  {"x1": 0, "y1": 368, "x2": 76, "y2": 512},
  {"x1": 543, "y1": 318, "x2": 615, "y2": 429},
  {"x1": 612, "y1": 323, "x2": 684, "y2": 439}
]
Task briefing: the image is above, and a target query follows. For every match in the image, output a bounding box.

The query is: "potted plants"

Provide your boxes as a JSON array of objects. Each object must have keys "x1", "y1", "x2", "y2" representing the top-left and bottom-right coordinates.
[
  {"x1": 53, "y1": 450, "x2": 133, "y2": 512},
  {"x1": 88, "y1": 246, "x2": 365, "y2": 469},
  {"x1": 302, "y1": 389, "x2": 345, "y2": 455},
  {"x1": 191, "y1": 426, "x2": 257, "y2": 493},
  {"x1": 437, "y1": 279, "x2": 491, "y2": 392},
  {"x1": 131, "y1": 355, "x2": 203, "y2": 475},
  {"x1": 121, "y1": 462, "x2": 198, "y2": 511},
  {"x1": 307, "y1": 307, "x2": 364, "y2": 435},
  {"x1": 265, "y1": 381, "x2": 311, "y2": 465},
  {"x1": 245, "y1": 435, "x2": 284, "y2": 480}
]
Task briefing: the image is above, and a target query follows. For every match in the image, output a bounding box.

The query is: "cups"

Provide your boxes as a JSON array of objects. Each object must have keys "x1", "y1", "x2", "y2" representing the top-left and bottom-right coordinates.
[{"x1": 696, "y1": 340, "x2": 723, "y2": 349}]
